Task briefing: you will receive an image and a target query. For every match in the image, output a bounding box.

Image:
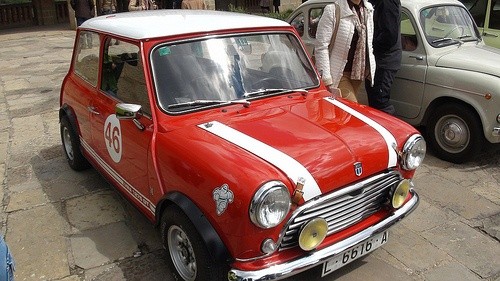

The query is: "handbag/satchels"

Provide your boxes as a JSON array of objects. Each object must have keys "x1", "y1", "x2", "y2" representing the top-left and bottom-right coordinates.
[{"x1": 311, "y1": 48, "x2": 331, "y2": 66}]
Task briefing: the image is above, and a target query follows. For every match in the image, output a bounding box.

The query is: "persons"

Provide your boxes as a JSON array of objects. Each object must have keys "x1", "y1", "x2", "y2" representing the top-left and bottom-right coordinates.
[
  {"x1": 314, "y1": 0, "x2": 376, "y2": 106},
  {"x1": 366, "y1": 0, "x2": 402, "y2": 115},
  {"x1": 69, "y1": 0, "x2": 208, "y2": 27},
  {"x1": 404, "y1": 36, "x2": 417, "y2": 52}
]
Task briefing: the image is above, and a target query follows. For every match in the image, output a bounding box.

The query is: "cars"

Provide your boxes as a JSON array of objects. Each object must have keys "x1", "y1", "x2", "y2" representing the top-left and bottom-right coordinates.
[
  {"x1": 261, "y1": 0, "x2": 499, "y2": 164},
  {"x1": 59, "y1": 9, "x2": 426, "y2": 281}
]
